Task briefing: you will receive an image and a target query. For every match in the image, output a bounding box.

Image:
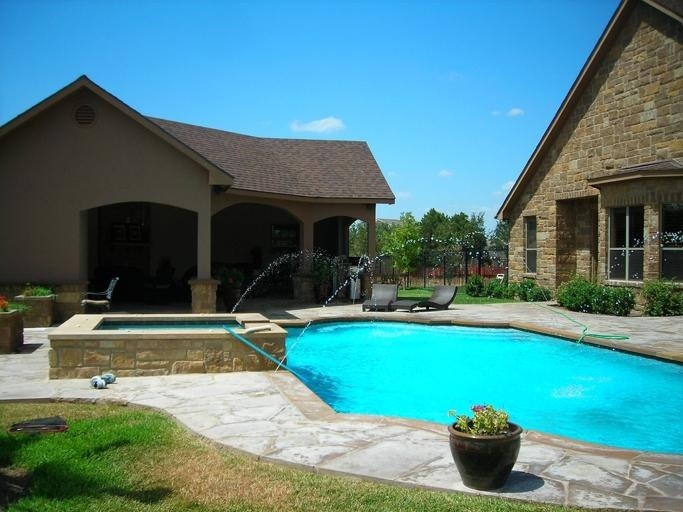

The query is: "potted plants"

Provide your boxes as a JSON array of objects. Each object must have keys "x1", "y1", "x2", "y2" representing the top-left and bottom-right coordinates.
[{"x1": 13, "y1": 281, "x2": 57, "y2": 328}]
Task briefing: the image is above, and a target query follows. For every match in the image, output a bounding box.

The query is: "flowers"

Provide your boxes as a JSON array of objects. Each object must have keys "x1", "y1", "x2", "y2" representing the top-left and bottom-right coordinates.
[
  {"x1": 445, "y1": 403, "x2": 511, "y2": 433},
  {"x1": 216, "y1": 267, "x2": 244, "y2": 288},
  {"x1": 0, "y1": 295, "x2": 31, "y2": 314}
]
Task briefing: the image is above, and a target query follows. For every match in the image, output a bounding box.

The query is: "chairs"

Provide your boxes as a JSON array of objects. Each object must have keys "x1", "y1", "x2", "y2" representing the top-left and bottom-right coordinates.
[
  {"x1": 392, "y1": 284, "x2": 459, "y2": 313},
  {"x1": 361, "y1": 282, "x2": 399, "y2": 312},
  {"x1": 78, "y1": 276, "x2": 121, "y2": 312}
]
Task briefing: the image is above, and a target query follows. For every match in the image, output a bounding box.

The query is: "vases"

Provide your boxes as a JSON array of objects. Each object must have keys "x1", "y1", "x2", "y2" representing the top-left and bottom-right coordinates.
[
  {"x1": 223, "y1": 287, "x2": 241, "y2": 312},
  {"x1": 448, "y1": 417, "x2": 523, "y2": 491}
]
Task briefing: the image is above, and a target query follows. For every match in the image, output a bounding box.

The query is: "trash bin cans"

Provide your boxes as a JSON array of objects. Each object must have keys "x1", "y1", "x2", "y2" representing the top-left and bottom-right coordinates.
[{"x1": 349, "y1": 277, "x2": 361, "y2": 304}]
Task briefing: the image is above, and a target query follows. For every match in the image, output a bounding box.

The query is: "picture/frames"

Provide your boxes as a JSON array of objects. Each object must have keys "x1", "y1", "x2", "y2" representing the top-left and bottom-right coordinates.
[
  {"x1": 126, "y1": 223, "x2": 142, "y2": 243},
  {"x1": 110, "y1": 221, "x2": 127, "y2": 242}
]
